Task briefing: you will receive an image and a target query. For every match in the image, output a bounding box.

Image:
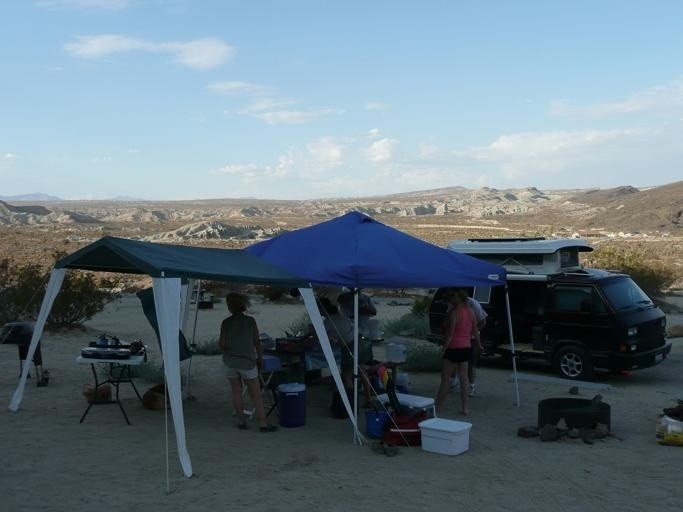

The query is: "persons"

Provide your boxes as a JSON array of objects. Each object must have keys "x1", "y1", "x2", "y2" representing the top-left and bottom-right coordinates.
[
  {"x1": 217, "y1": 291, "x2": 280, "y2": 433},
  {"x1": 303, "y1": 296, "x2": 367, "y2": 419},
  {"x1": 433, "y1": 289, "x2": 486, "y2": 416},
  {"x1": 444, "y1": 296, "x2": 487, "y2": 397},
  {"x1": 337, "y1": 283, "x2": 378, "y2": 326}
]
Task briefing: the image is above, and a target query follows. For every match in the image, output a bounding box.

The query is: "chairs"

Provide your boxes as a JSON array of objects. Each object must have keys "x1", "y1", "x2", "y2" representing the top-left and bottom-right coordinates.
[{"x1": 232, "y1": 368, "x2": 278, "y2": 421}]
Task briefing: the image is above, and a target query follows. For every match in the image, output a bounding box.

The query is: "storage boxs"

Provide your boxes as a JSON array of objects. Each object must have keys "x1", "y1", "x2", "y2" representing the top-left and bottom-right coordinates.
[
  {"x1": 417, "y1": 418, "x2": 472, "y2": 455},
  {"x1": 378, "y1": 393, "x2": 435, "y2": 419}
]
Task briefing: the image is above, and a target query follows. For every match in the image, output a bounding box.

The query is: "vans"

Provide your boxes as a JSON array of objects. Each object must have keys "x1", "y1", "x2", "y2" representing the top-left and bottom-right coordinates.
[{"x1": 425, "y1": 268, "x2": 671, "y2": 380}]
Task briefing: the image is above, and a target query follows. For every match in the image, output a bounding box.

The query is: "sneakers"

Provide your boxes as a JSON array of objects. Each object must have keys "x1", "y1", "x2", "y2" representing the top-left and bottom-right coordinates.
[
  {"x1": 238, "y1": 422, "x2": 247, "y2": 429},
  {"x1": 260, "y1": 423, "x2": 278, "y2": 432},
  {"x1": 450, "y1": 382, "x2": 460, "y2": 392},
  {"x1": 469, "y1": 388, "x2": 476, "y2": 397}
]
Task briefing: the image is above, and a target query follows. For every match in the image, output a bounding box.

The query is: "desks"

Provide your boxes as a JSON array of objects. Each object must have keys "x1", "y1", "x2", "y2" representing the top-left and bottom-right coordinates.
[{"x1": 76, "y1": 345, "x2": 150, "y2": 425}]
